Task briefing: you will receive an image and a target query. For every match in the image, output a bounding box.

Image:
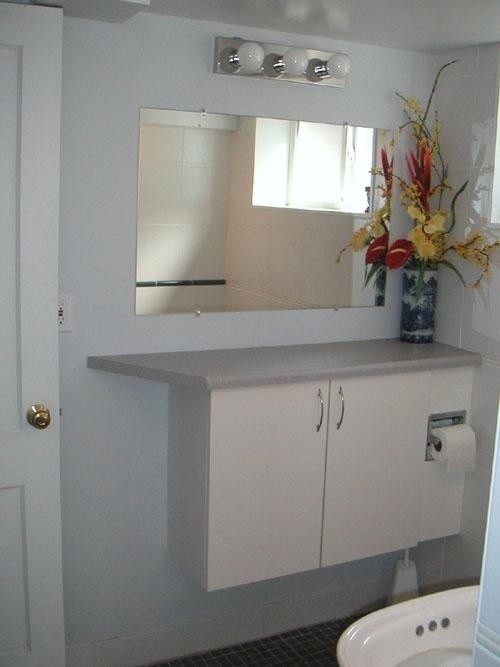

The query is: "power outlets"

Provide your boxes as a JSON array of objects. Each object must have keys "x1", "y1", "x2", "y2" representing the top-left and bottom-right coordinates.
[{"x1": 59, "y1": 294, "x2": 75, "y2": 331}]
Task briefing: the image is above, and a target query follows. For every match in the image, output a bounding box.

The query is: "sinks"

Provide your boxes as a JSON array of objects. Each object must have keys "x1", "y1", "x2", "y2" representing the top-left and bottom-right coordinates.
[{"x1": 337, "y1": 583, "x2": 481, "y2": 667}]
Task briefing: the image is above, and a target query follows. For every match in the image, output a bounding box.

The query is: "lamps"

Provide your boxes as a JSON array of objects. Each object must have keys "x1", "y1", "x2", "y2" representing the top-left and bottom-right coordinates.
[{"x1": 213, "y1": 35, "x2": 354, "y2": 89}]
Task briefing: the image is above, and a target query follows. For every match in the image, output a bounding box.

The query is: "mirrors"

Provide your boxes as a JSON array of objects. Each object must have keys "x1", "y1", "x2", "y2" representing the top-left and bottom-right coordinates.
[{"x1": 134, "y1": 107, "x2": 396, "y2": 315}]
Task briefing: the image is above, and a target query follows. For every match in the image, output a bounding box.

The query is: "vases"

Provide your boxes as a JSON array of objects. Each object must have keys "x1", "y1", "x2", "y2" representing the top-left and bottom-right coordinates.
[
  {"x1": 374, "y1": 258, "x2": 387, "y2": 305},
  {"x1": 399, "y1": 261, "x2": 439, "y2": 343}
]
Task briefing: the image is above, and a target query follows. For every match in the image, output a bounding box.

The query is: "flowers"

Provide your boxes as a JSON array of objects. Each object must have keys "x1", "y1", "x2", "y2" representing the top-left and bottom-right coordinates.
[
  {"x1": 368, "y1": 56, "x2": 500, "y2": 306},
  {"x1": 335, "y1": 129, "x2": 396, "y2": 288}
]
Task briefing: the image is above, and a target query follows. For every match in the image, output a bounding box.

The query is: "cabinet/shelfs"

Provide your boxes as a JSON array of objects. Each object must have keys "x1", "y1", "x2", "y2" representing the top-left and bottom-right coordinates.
[{"x1": 167, "y1": 365, "x2": 476, "y2": 593}]
